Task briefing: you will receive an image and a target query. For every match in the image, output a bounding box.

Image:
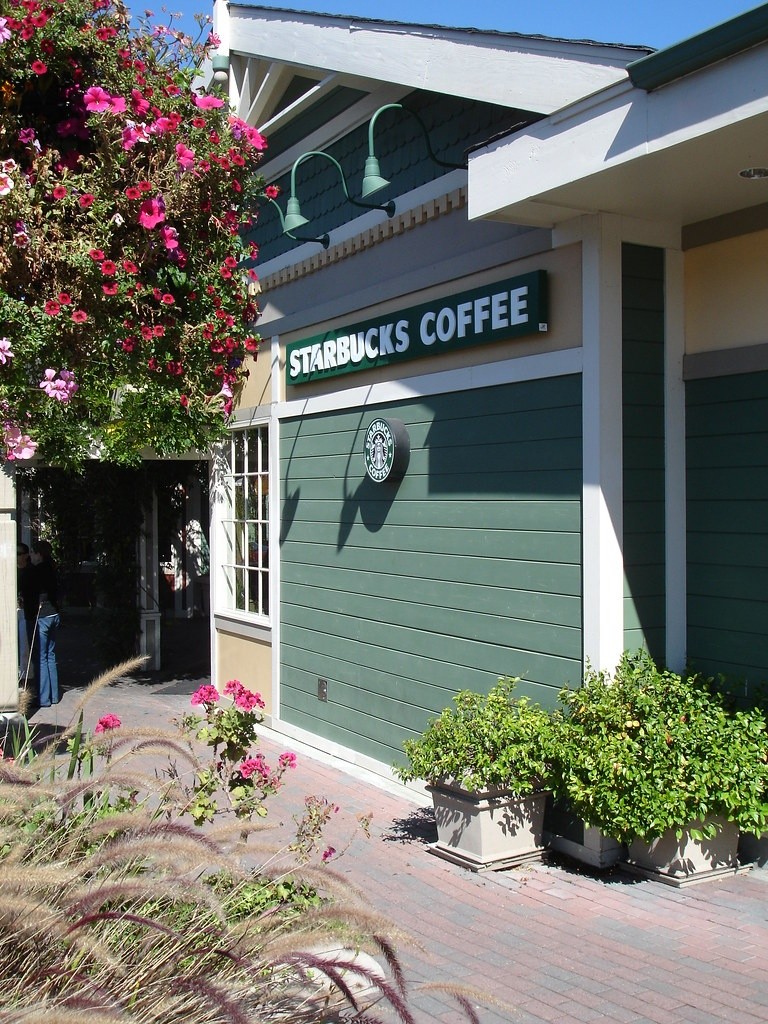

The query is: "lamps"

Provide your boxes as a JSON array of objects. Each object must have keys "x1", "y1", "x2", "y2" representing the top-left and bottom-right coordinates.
[
  {"x1": 282, "y1": 152, "x2": 396, "y2": 234},
  {"x1": 362, "y1": 104, "x2": 468, "y2": 200}
]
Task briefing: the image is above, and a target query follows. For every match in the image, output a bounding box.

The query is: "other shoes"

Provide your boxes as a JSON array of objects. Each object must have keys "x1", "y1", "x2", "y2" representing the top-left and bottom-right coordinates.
[
  {"x1": 19, "y1": 678, "x2": 34, "y2": 687},
  {"x1": 32, "y1": 701, "x2": 51, "y2": 707}
]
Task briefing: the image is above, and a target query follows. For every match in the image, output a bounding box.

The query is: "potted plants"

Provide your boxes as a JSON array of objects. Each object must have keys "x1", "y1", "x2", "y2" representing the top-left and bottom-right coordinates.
[
  {"x1": 537, "y1": 638, "x2": 768, "y2": 889},
  {"x1": 388, "y1": 670, "x2": 559, "y2": 873}
]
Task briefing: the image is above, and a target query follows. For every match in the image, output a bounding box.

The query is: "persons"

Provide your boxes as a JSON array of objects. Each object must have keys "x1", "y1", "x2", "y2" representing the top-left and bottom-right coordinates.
[{"x1": 17, "y1": 540, "x2": 60, "y2": 707}]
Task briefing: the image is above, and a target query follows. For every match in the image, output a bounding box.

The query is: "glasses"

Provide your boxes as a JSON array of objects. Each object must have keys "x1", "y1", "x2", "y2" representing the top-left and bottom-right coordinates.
[{"x1": 17, "y1": 552, "x2": 26, "y2": 556}]
